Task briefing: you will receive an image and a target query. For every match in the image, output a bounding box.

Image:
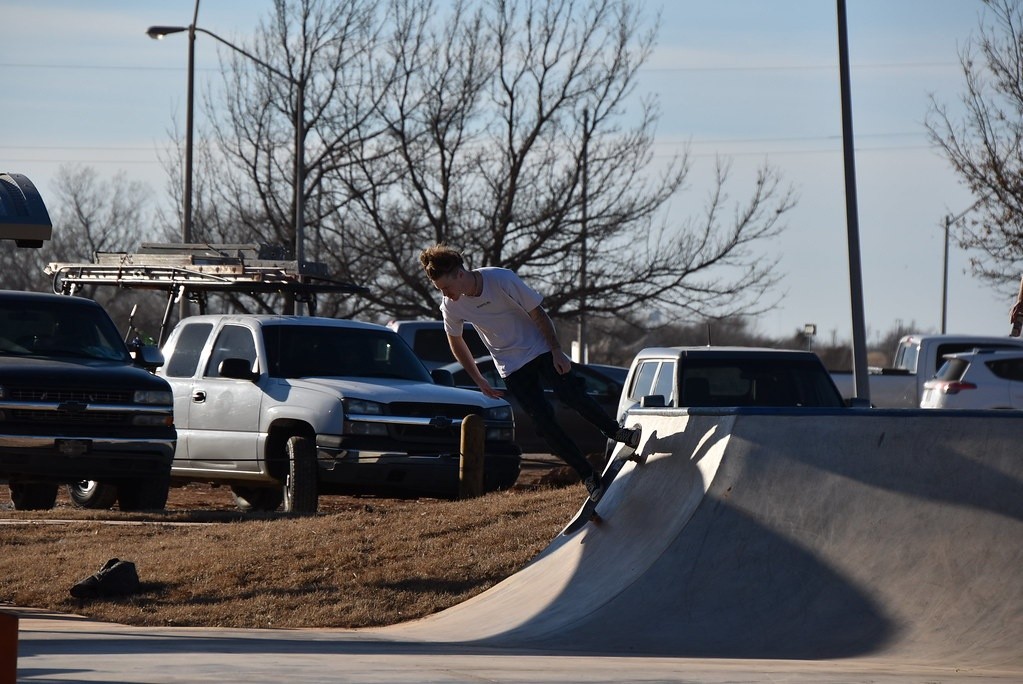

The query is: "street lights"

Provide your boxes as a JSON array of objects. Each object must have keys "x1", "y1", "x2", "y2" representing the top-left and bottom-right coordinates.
[
  {"x1": 935, "y1": 176, "x2": 1015, "y2": 335},
  {"x1": 142, "y1": 12, "x2": 314, "y2": 320}
]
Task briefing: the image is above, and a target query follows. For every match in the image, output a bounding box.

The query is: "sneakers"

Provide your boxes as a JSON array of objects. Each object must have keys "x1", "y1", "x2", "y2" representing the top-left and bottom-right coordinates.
[
  {"x1": 584, "y1": 469, "x2": 604, "y2": 502},
  {"x1": 612, "y1": 428, "x2": 641, "y2": 446}
]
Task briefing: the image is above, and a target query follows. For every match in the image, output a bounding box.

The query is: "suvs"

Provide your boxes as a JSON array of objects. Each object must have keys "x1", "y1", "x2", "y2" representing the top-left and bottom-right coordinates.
[
  {"x1": 616, "y1": 321, "x2": 875, "y2": 494},
  {"x1": 917, "y1": 346, "x2": 1023, "y2": 413},
  {"x1": 1, "y1": 167, "x2": 178, "y2": 520}
]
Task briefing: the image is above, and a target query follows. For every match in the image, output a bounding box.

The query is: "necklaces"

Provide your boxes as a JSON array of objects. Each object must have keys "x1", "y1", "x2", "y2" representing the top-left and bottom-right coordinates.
[{"x1": 465, "y1": 271, "x2": 478, "y2": 298}]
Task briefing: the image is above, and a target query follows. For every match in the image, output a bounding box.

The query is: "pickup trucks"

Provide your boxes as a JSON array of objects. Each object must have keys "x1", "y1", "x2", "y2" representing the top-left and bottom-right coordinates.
[
  {"x1": 813, "y1": 333, "x2": 1023, "y2": 416},
  {"x1": 43, "y1": 238, "x2": 525, "y2": 519}
]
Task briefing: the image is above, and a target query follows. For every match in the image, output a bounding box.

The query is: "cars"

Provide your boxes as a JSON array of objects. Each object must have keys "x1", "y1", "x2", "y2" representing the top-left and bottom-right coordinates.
[{"x1": 424, "y1": 354, "x2": 633, "y2": 466}]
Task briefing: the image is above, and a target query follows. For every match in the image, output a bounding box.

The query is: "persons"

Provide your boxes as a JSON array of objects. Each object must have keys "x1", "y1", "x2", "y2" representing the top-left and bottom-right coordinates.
[{"x1": 422, "y1": 247, "x2": 641, "y2": 499}]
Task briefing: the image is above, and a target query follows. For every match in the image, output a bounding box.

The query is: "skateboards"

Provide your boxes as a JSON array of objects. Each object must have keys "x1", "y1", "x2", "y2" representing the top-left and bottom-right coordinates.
[{"x1": 563, "y1": 423, "x2": 643, "y2": 535}]
